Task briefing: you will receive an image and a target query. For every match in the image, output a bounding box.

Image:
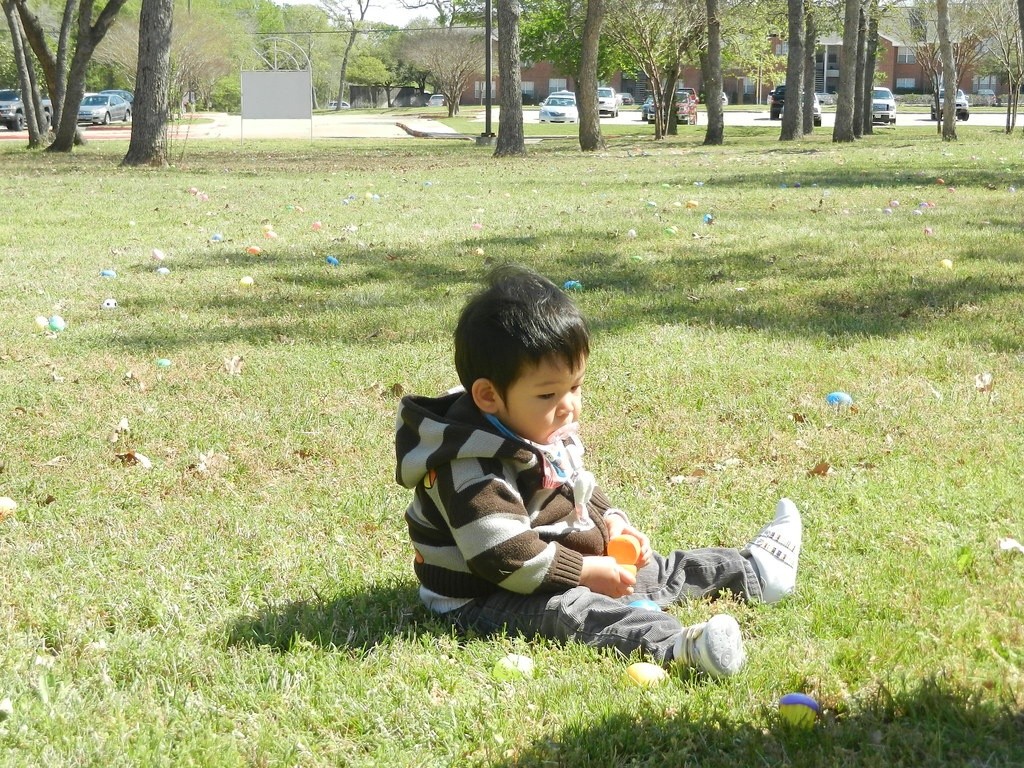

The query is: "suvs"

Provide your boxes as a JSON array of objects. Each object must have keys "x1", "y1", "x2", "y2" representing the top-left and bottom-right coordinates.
[
  {"x1": 594, "y1": 87, "x2": 620, "y2": 118},
  {"x1": 931, "y1": 88, "x2": 970, "y2": 120}
]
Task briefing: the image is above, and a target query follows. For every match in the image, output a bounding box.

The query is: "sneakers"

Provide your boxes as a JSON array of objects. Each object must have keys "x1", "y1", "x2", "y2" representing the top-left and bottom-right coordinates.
[
  {"x1": 739, "y1": 496, "x2": 803, "y2": 605},
  {"x1": 672, "y1": 614, "x2": 746, "y2": 681}
]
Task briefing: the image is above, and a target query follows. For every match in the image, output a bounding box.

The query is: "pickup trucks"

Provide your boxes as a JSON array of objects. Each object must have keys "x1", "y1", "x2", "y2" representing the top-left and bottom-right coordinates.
[{"x1": 0, "y1": 88, "x2": 52, "y2": 131}]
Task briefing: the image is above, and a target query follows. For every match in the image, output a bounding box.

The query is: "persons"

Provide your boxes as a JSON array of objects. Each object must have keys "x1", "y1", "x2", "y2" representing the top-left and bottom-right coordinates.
[{"x1": 393, "y1": 266, "x2": 802, "y2": 680}]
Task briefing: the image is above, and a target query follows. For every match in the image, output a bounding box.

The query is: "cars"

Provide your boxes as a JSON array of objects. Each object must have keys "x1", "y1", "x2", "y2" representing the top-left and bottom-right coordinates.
[
  {"x1": 641, "y1": 96, "x2": 654, "y2": 120},
  {"x1": 424, "y1": 94, "x2": 445, "y2": 107},
  {"x1": 648, "y1": 92, "x2": 697, "y2": 126},
  {"x1": 799, "y1": 91, "x2": 821, "y2": 126},
  {"x1": 538, "y1": 94, "x2": 579, "y2": 124},
  {"x1": 871, "y1": 87, "x2": 897, "y2": 123},
  {"x1": 329, "y1": 102, "x2": 349, "y2": 108},
  {"x1": 100, "y1": 89, "x2": 134, "y2": 105},
  {"x1": 618, "y1": 93, "x2": 634, "y2": 105},
  {"x1": 722, "y1": 92, "x2": 728, "y2": 106},
  {"x1": 78, "y1": 94, "x2": 131, "y2": 125},
  {"x1": 676, "y1": 88, "x2": 699, "y2": 104},
  {"x1": 977, "y1": 89, "x2": 996, "y2": 105},
  {"x1": 549, "y1": 90, "x2": 576, "y2": 98},
  {"x1": 768, "y1": 84, "x2": 787, "y2": 120}
]
